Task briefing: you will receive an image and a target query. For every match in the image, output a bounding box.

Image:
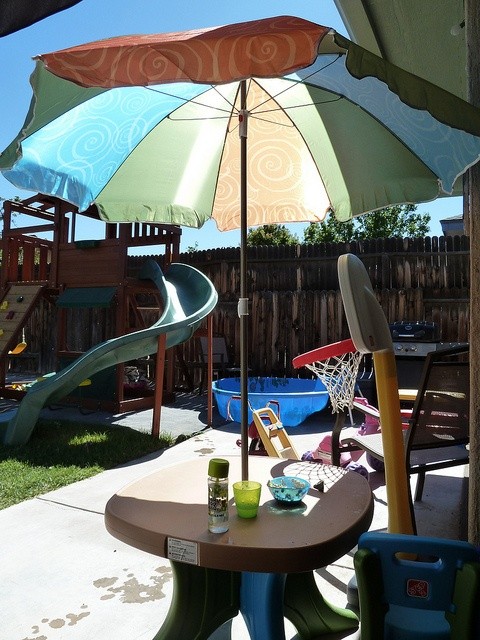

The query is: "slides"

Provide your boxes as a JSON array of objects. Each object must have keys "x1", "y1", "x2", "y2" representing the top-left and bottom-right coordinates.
[{"x1": 4, "y1": 258, "x2": 219, "y2": 454}]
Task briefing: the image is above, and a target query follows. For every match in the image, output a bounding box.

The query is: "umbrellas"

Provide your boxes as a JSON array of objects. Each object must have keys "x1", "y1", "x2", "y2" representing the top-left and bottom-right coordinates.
[{"x1": 1, "y1": 15, "x2": 478, "y2": 483}]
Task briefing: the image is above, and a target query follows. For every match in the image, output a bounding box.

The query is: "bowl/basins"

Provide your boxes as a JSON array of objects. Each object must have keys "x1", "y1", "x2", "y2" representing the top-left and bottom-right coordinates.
[{"x1": 267, "y1": 476, "x2": 311, "y2": 506}]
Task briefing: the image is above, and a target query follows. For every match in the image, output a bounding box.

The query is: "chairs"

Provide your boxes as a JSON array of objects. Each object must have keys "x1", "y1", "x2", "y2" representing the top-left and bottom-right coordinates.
[
  {"x1": 328, "y1": 342, "x2": 470, "y2": 503},
  {"x1": 353, "y1": 531, "x2": 479, "y2": 640},
  {"x1": 199, "y1": 336, "x2": 258, "y2": 399}
]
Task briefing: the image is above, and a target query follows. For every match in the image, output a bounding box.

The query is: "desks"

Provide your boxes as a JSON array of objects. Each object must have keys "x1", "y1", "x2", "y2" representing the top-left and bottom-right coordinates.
[{"x1": 104, "y1": 453, "x2": 376, "y2": 640}]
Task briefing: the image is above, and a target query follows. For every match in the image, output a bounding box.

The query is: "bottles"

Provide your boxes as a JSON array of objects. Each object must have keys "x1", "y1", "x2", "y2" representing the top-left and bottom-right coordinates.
[{"x1": 208, "y1": 458, "x2": 230, "y2": 534}]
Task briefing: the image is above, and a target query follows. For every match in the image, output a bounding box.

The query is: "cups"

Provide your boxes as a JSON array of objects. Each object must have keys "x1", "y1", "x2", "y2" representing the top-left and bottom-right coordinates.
[{"x1": 233, "y1": 481, "x2": 262, "y2": 520}]
